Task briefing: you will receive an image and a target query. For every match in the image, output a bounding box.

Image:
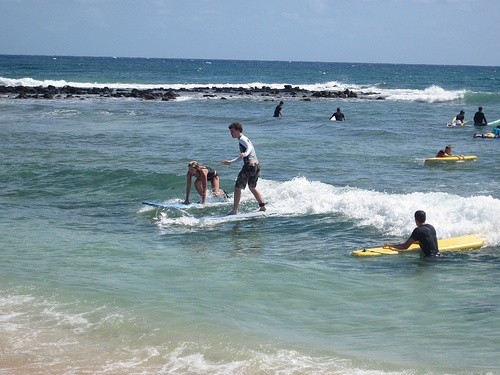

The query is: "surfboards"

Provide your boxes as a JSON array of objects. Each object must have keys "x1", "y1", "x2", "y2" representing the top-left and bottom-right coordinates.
[
  {"x1": 200, "y1": 209, "x2": 280, "y2": 221},
  {"x1": 330, "y1": 116, "x2": 336, "y2": 120},
  {"x1": 453, "y1": 116, "x2": 464, "y2": 126},
  {"x1": 487, "y1": 119, "x2": 500, "y2": 126},
  {"x1": 142, "y1": 200, "x2": 237, "y2": 209},
  {"x1": 426, "y1": 155, "x2": 477, "y2": 161},
  {"x1": 351, "y1": 235, "x2": 483, "y2": 257}
]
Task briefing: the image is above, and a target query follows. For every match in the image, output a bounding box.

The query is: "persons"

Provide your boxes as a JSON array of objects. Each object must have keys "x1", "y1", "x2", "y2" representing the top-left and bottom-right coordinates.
[
  {"x1": 182, "y1": 161, "x2": 229, "y2": 204},
  {"x1": 474, "y1": 106, "x2": 487, "y2": 126},
  {"x1": 329, "y1": 108, "x2": 346, "y2": 121},
  {"x1": 456, "y1": 111, "x2": 465, "y2": 122},
  {"x1": 436, "y1": 145, "x2": 451, "y2": 157},
  {"x1": 222, "y1": 122, "x2": 266, "y2": 216},
  {"x1": 474, "y1": 124, "x2": 500, "y2": 138},
  {"x1": 384, "y1": 211, "x2": 440, "y2": 256},
  {"x1": 273, "y1": 101, "x2": 284, "y2": 117}
]
